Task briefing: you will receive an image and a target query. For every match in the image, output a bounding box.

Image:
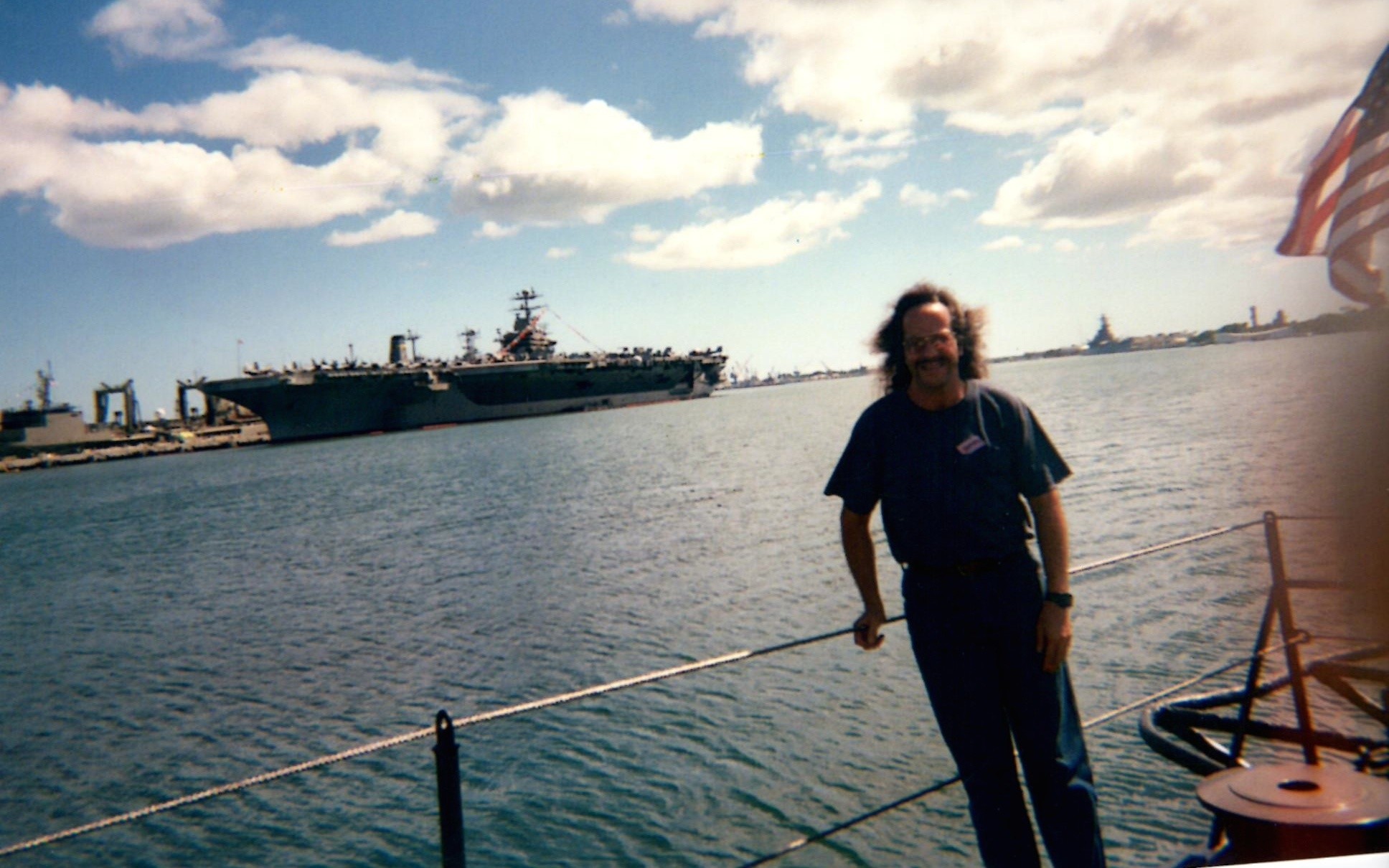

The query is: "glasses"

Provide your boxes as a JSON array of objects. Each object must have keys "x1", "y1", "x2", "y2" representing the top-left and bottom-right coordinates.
[{"x1": 901, "y1": 330, "x2": 958, "y2": 356}]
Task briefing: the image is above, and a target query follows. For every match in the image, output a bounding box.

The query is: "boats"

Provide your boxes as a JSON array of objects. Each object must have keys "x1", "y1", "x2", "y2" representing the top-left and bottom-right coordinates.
[{"x1": 191, "y1": 288, "x2": 728, "y2": 444}]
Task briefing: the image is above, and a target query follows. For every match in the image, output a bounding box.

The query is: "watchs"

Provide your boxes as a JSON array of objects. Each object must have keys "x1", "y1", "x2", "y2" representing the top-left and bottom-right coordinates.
[{"x1": 1044, "y1": 590, "x2": 1072, "y2": 607}]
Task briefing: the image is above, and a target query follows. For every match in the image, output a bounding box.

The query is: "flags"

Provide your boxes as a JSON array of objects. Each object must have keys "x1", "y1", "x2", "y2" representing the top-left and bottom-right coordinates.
[{"x1": 1275, "y1": 43, "x2": 1389, "y2": 307}]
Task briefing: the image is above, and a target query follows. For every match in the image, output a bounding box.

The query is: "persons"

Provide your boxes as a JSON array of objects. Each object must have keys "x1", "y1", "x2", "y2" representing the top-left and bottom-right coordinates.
[{"x1": 822, "y1": 284, "x2": 1110, "y2": 868}]
